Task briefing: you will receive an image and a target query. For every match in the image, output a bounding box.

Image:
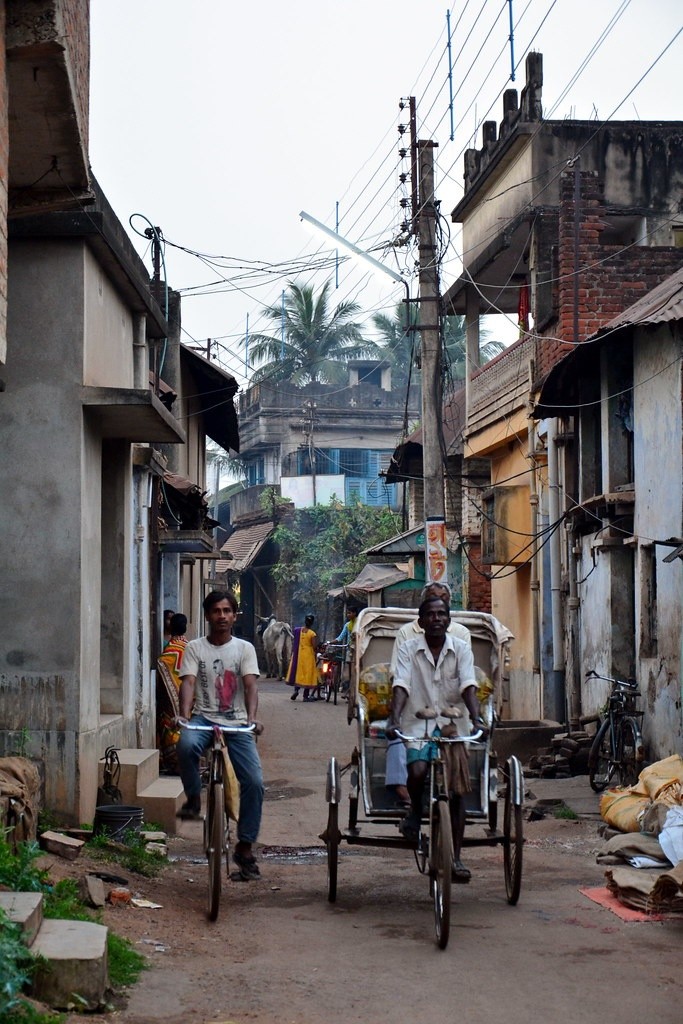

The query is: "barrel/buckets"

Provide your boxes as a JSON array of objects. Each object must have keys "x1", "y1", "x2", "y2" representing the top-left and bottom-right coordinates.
[{"x1": 94, "y1": 805, "x2": 144, "y2": 844}]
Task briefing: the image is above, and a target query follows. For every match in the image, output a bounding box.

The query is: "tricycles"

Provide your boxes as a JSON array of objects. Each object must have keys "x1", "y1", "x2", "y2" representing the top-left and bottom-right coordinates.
[{"x1": 320, "y1": 607, "x2": 527, "y2": 950}]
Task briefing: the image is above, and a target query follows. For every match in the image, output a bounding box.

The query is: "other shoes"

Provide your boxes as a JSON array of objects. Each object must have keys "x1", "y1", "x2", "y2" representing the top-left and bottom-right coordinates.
[
  {"x1": 232, "y1": 851, "x2": 259, "y2": 877},
  {"x1": 400, "y1": 818, "x2": 419, "y2": 839},
  {"x1": 311, "y1": 697, "x2": 325, "y2": 700},
  {"x1": 303, "y1": 697, "x2": 314, "y2": 702},
  {"x1": 451, "y1": 859, "x2": 470, "y2": 882},
  {"x1": 291, "y1": 692, "x2": 299, "y2": 701},
  {"x1": 176, "y1": 802, "x2": 202, "y2": 818}
]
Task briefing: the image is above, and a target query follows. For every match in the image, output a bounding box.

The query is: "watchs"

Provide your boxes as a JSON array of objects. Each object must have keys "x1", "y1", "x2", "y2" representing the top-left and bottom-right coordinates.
[{"x1": 473, "y1": 716, "x2": 484, "y2": 724}]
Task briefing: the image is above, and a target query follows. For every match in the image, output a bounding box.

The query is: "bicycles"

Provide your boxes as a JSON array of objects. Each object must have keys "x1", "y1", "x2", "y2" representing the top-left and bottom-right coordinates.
[
  {"x1": 315, "y1": 641, "x2": 349, "y2": 705},
  {"x1": 176, "y1": 717, "x2": 259, "y2": 922},
  {"x1": 585, "y1": 670, "x2": 646, "y2": 794}
]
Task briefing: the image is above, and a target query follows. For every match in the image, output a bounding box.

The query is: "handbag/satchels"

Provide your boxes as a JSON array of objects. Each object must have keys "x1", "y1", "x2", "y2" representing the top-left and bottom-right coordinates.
[{"x1": 215, "y1": 727, "x2": 240, "y2": 822}]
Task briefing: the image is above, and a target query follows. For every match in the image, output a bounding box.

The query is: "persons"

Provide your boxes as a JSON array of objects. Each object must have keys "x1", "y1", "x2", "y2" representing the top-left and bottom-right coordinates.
[
  {"x1": 384, "y1": 581, "x2": 489, "y2": 880},
  {"x1": 323, "y1": 606, "x2": 357, "y2": 693},
  {"x1": 156, "y1": 591, "x2": 263, "y2": 879},
  {"x1": 285, "y1": 615, "x2": 318, "y2": 704}
]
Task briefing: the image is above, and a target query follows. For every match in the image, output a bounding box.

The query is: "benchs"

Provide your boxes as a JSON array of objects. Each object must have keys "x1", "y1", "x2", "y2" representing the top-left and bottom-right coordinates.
[{"x1": 360, "y1": 662, "x2": 494, "y2": 737}]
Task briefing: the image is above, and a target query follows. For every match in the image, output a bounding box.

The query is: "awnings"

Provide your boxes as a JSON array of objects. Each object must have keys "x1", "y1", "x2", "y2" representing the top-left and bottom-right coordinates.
[{"x1": 209, "y1": 523, "x2": 275, "y2": 576}]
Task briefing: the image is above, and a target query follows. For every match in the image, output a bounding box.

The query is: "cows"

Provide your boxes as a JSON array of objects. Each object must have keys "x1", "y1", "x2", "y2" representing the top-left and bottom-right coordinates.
[{"x1": 255, "y1": 613, "x2": 293, "y2": 681}]
